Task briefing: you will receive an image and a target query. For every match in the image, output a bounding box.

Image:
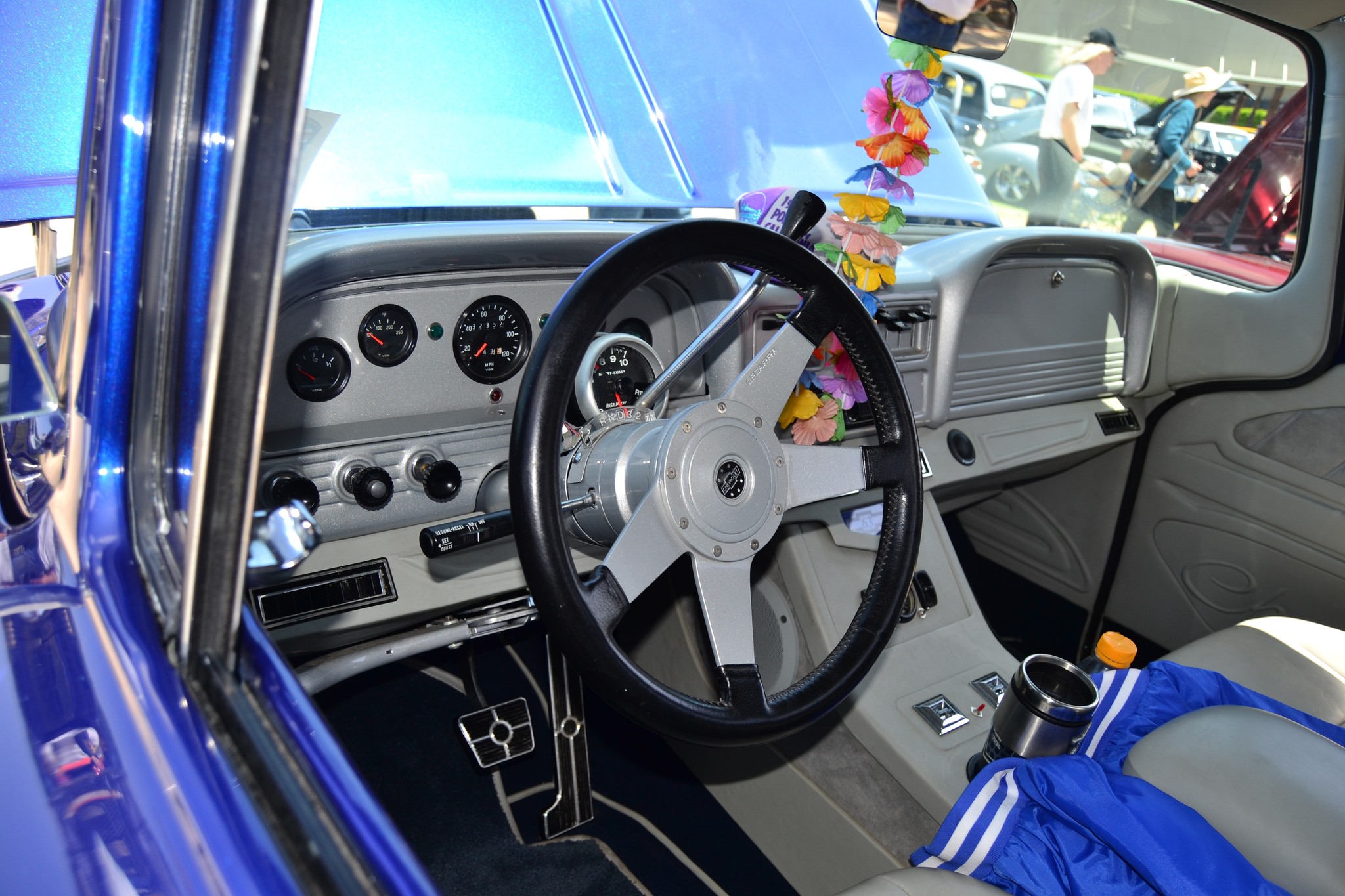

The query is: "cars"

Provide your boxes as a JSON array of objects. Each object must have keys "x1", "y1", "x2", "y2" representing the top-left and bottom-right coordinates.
[{"x1": 921, "y1": 53, "x2": 1287, "y2": 219}]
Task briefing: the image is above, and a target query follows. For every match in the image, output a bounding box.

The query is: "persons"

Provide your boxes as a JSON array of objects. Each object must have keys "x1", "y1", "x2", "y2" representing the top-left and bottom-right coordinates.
[
  {"x1": 1026, "y1": 27, "x2": 1125, "y2": 228},
  {"x1": 895, "y1": 1, "x2": 990, "y2": 50},
  {"x1": 1119, "y1": 66, "x2": 1234, "y2": 237}
]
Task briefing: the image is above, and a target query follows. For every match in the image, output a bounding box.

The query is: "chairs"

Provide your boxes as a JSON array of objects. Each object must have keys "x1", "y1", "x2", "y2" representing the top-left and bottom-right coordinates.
[{"x1": 1134, "y1": 609, "x2": 1345, "y2": 735}]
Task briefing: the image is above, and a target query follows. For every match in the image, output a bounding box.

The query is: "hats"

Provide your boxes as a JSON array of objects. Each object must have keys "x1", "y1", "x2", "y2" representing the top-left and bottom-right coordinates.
[
  {"x1": 1084, "y1": 27, "x2": 1124, "y2": 57},
  {"x1": 1172, "y1": 66, "x2": 1232, "y2": 101}
]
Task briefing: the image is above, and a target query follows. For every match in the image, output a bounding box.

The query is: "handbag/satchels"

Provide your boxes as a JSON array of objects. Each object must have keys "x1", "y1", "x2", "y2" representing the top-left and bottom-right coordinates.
[{"x1": 1128, "y1": 139, "x2": 1161, "y2": 181}]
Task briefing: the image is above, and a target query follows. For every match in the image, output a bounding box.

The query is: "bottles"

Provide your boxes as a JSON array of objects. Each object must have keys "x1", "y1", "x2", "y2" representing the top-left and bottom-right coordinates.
[{"x1": 1077, "y1": 631, "x2": 1137, "y2": 676}]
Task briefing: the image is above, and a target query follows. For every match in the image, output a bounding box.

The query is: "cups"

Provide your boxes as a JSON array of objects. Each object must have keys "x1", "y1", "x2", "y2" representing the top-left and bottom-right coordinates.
[{"x1": 966, "y1": 653, "x2": 1100, "y2": 782}]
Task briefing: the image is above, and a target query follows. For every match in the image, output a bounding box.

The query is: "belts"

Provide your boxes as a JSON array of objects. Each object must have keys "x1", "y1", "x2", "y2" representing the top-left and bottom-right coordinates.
[{"x1": 909, "y1": 0, "x2": 957, "y2": 25}]
